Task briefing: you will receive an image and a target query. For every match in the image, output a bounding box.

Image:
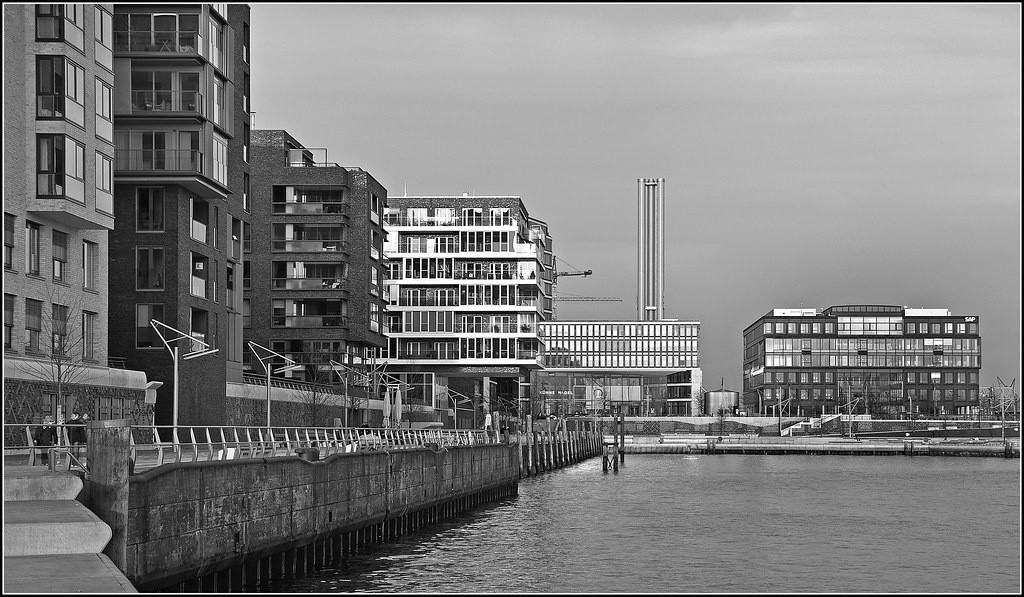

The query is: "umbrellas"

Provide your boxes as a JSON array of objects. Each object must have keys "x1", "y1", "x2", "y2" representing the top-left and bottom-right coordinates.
[{"x1": 382, "y1": 389, "x2": 402, "y2": 439}]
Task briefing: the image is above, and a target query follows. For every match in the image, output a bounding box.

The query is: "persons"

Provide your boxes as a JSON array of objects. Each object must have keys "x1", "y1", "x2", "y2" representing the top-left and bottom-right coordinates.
[
  {"x1": 359, "y1": 421, "x2": 371, "y2": 436},
  {"x1": 34, "y1": 413, "x2": 92, "y2": 467}
]
